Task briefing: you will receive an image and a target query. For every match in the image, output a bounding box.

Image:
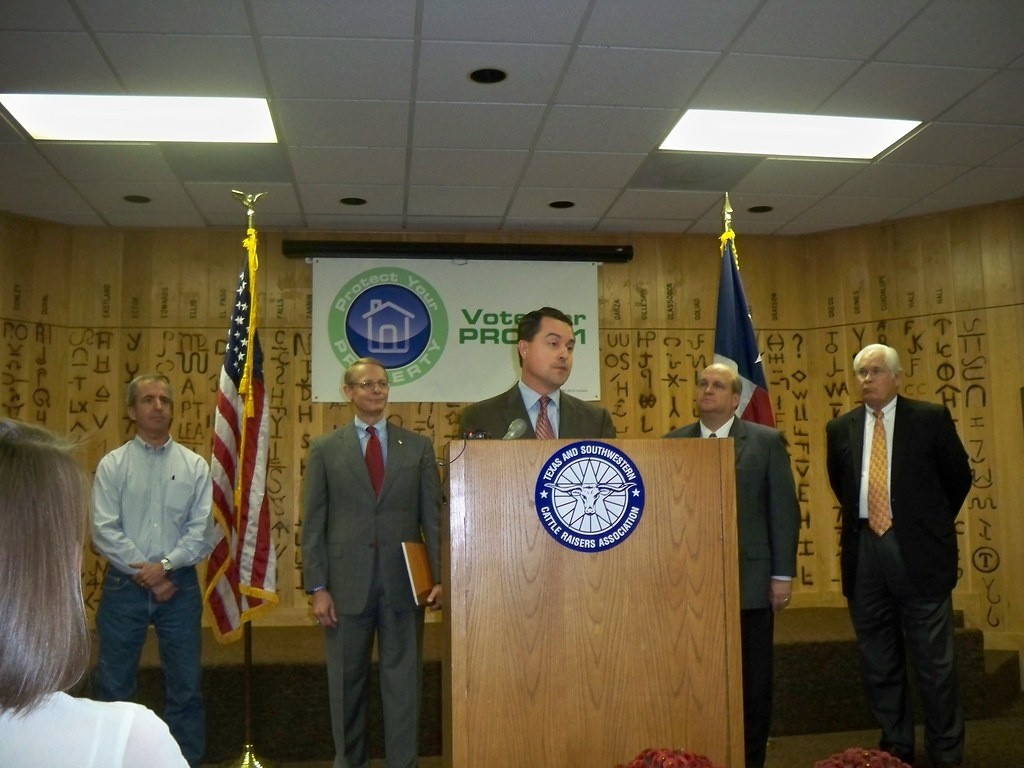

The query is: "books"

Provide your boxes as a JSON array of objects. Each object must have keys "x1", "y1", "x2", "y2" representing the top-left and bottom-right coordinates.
[{"x1": 401, "y1": 542, "x2": 436, "y2": 606}]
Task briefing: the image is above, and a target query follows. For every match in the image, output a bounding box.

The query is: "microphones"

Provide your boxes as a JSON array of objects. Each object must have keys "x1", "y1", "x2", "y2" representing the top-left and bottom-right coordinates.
[{"x1": 502, "y1": 419, "x2": 527, "y2": 440}]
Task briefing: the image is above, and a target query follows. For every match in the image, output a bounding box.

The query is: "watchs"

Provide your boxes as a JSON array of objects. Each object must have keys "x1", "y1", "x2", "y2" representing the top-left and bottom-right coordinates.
[{"x1": 161, "y1": 559, "x2": 173, "y2": 574}]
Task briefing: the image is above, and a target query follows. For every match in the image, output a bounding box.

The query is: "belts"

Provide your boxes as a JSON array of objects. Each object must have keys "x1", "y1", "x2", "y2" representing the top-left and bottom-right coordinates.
[{"x1": 859, "y1": 520, "x2": 896, "y2": 529}]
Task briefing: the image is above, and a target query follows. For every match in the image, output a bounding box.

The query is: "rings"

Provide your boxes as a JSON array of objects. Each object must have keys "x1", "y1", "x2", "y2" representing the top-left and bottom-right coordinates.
[
  {"x1": 317, "y1": 620, "x2": 320, "y2": 624},
  {"x1": 141, "y1": 582, "x2": 144, "y2": 587},
  {"x1": 784, "y1": 599, "x2": 788, "y2": 601}
]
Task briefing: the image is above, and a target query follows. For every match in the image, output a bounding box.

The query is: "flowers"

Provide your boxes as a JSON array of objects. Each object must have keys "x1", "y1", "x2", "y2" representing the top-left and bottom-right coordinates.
[
  {"x1": 813, "y1": 748, "x2": 912, "y2": 768},
  {"x1": 614, "y1": 748, "x2": 715, "y2": 768}
]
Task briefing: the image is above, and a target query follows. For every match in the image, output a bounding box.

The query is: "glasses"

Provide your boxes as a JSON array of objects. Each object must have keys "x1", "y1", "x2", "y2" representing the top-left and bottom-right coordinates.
[{"x1": 350, "y1": 381, "x2": 391, "y2": 389}]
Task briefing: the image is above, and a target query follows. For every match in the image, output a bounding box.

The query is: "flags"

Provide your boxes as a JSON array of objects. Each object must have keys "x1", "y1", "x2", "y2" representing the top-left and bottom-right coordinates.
[
  {"x1": 714, "y1": 235, "x2": 774, "y2": 428},
  {"x1": 196, "y1": 226, "x2": 279, "y2": 644}
]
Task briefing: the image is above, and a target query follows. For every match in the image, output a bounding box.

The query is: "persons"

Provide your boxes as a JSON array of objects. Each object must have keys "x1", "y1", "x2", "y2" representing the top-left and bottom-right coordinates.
[
  {"x1": 0, "y1": 417, "x2": 191, "y2": 768},
  {"x1": 301, "y1": 358, "x2": 443, "y2": 768},
  {"x1": 458, "y1": 306, "x2": 616, "y2": 440},
  {"x1": 89, "y1": 373, "x2": 216, "y2": 768},
  {"x1": 826, "y1": 343, "x2": 972, "y2": 768},
  {"x1": 655, "y1": 363, "x2": 803, "y2": 768}
]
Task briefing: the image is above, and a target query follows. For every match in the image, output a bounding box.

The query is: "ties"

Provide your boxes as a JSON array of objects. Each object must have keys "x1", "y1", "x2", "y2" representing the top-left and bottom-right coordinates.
[
  {"x1": 365, "y1": 426, "x2": 384, "y2": 497},
  {"x1": 709, "y1": 433, "x2": 717, "y2": 437},
  {"x1": 536, "y1": 397, "x2": 555, "y2": 440},
  {"x1": 867, "y1": 411, "x2": 892, "y2": 536}
]
